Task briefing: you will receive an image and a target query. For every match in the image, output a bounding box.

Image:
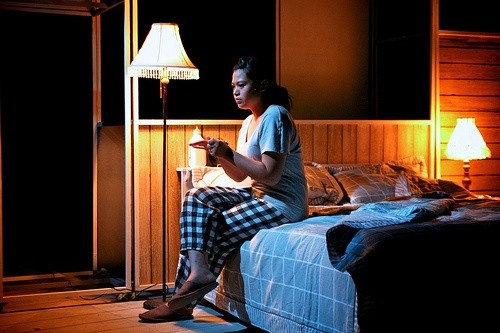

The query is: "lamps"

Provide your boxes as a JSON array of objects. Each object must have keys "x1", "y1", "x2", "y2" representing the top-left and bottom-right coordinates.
[
  {"x1": 444, "y1": 116, "x2": 491, "y2": 191},
  {"x1": 123, "y1": 22, "x2": 199, "y2": 310}
]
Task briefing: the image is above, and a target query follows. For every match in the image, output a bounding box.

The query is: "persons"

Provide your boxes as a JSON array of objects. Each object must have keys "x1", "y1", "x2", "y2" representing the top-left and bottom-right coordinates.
[{"x1": 139, "y1": 58, "x2": 310, "y2": 323}]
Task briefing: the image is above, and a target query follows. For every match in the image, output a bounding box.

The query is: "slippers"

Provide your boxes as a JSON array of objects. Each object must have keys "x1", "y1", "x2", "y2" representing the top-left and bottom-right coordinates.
[
  {"x1": 139, "y1": 303, "x2": 194, "y2": 322},
  {"x1": 167, "y1": 280, "x2": 219, "y2": 310}
]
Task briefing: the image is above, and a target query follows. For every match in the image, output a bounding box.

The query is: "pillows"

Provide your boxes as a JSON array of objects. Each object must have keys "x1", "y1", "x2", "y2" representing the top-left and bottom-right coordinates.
[
  {"x1": 329, "y1": 155, "x2": 428, "y2": 203},
  {"x1": 190, "y1": 160, "x2": 343, "y2": 206}
]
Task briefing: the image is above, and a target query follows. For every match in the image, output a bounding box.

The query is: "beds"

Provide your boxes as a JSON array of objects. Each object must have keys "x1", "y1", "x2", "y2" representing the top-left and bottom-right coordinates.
[{"x1": 175, "y1": 161, "x2": 500, "y2": 332}]
